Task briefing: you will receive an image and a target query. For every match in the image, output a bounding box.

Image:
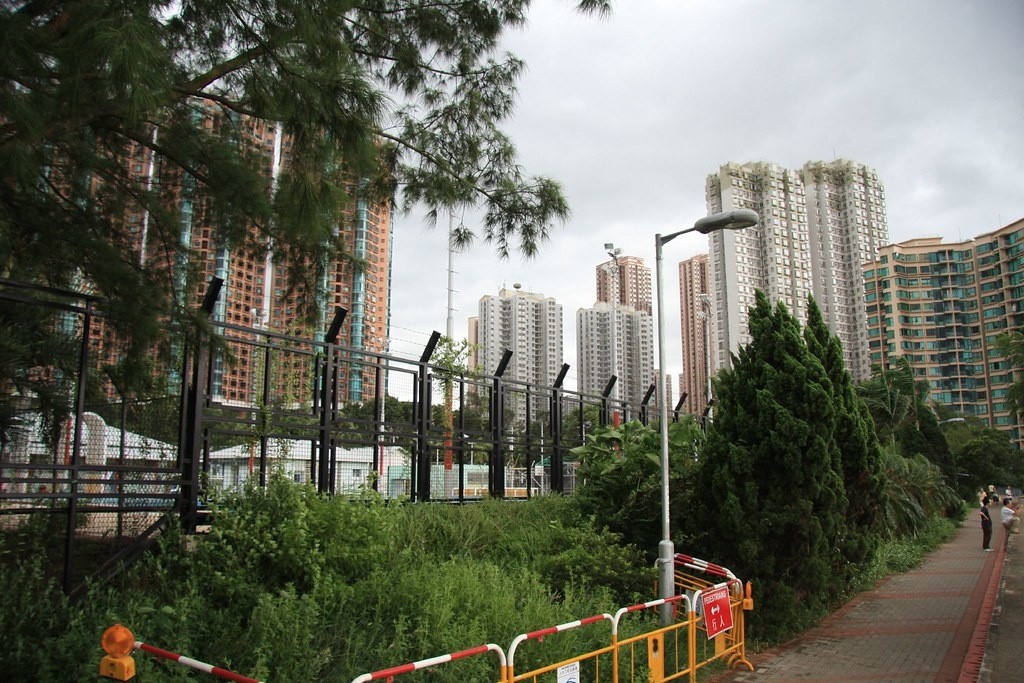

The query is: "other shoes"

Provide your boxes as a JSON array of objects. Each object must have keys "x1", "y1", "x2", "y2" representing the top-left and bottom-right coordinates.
[
  {"x1": 984, "y1": 548, "x2": 995, "y2": 552},
  {"x1": 1012, "y1": 530, "x2": 1020, "y2": 534}
]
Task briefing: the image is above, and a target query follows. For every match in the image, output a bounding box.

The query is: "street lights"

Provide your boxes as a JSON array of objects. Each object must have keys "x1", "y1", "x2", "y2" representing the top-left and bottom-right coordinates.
[{"x1": 651, "y1": 208, "x2": 758, "y2": 624}]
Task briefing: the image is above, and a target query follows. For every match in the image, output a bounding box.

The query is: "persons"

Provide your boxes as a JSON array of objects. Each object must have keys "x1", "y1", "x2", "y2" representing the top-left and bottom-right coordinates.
[
  {"x1": 976, "y1": 484, "x2": 1012, "y2": 508},
  {"x1": 980, "y1": 496, "x2": 995, "y2": 552},
  {"x1": 1000, "y1": 497, "x2": 1020, "y2": 551}
]
